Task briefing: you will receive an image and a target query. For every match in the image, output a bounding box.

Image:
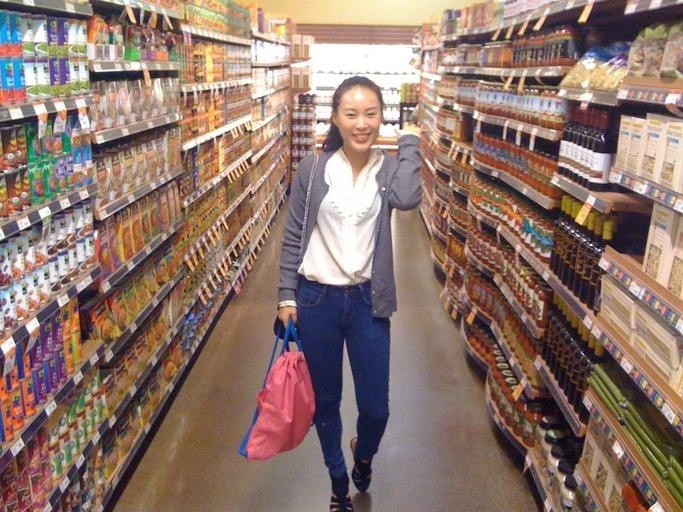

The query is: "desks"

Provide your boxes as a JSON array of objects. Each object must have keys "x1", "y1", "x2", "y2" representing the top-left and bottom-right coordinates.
[{"x1": 397, "y1": 102, "x2": 417, "y2": 130}]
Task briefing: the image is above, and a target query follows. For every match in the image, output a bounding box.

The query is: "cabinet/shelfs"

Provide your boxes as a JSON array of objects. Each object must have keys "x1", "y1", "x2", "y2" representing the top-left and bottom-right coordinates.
[
  {"x1": 312, "y1": 70, "x2": 417, "y2": 158},
  {"x1": 250, "y1": 24, "x2": 311, "y2": 270},
  {"x1": 89, "y1": 2, "x2": 186, "y2": 512},
  {"x1": 0, "y1": 0, "x2": 100, "y2": 511},
  {"x1": 181, "y1": 24, "x2": 250, "y2": 373}
]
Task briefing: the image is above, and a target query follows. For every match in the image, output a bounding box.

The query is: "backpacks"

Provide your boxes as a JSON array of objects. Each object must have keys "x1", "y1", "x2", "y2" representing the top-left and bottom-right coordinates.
[{"x1": 238, "y1": 317, "x2": 316, "y2": 461}]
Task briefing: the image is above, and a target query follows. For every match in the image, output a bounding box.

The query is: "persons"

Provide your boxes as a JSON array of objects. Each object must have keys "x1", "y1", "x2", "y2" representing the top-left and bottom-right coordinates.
[{"x1": 278, "y1": 76, "x2": 428, "y2": 512}]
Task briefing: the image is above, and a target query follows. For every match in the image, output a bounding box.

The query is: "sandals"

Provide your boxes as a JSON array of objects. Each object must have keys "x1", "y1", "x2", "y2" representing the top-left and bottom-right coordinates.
[
  {"x1": 330, "y1": 493, "x2": 354, "y2": 512},
  {"x1": 350, "y1": 437, "x2": 372, "y2": 492}
]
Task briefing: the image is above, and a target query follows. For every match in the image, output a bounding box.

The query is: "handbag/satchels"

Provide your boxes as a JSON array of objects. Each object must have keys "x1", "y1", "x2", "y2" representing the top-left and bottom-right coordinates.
[{"x1": 274, "y1": 315, "x2": 300, "y2": 342}]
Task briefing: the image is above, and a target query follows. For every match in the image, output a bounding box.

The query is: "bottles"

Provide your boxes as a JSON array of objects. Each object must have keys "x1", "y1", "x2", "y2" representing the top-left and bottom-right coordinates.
[
  {"x1": 466, "y1": 40, "x2": 512, "y2": 68},
  {"x1": 470, "y1": 170, "x2": 555, "y2": 264},
  {"x1": 541, "y1": 291, "x2": 604, "y2": 425},
  {"x1": 558, "y1": 104, "x2": 630, "y2": 193},
  {"x1": 449, "y1": 193, "x2": 472, "y2": 230},
  {"x1": 467, "y1": 222, "x2": 553, "y2": 328},
  {"x1": 439, "y1": 75, "x2": 458, "y2": 100},
  {"x1": 457, "y1": 79, "x2": 475, "y2": 106},
  {"x1": 466, "y1": 268, "x2": 545, "y2": 390},
  {"x1": 475, "y1": 80, "x2": 570, "y2": 131},
  {"x1": 550, "y1": 195, "x2": 626, "y2": 317},
  {"x1": 510, "y1": 23, "x2": 619, "y2": 65},
  {"x1": 472, "y1": 127, "x2": 563, "y2": 199}
]
